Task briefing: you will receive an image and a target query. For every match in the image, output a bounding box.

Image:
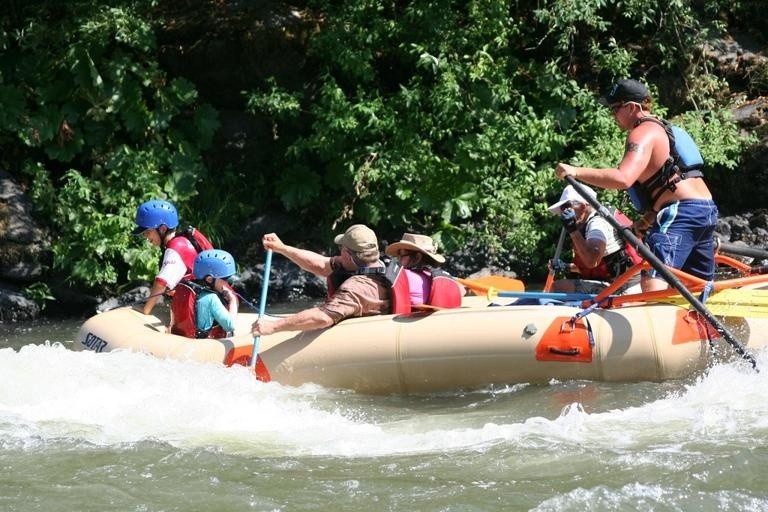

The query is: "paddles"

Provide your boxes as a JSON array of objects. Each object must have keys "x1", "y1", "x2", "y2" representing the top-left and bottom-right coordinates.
[
  {"x1": 497, "y1": 286, "x2": 767, "y2": 318},
  {"x1": 452, "y1": 272, "x2": 525, "y2": 297}
]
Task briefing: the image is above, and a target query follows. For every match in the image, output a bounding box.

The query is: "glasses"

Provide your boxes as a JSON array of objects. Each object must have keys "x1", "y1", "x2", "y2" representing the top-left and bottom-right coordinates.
[
  {"x1": 609, "y1": 104, "x2": 622, "y2": 116},
  {"x1": 397, "y1": 254, "x2": 409, "y2": 260},
  {"x1": 560, "y1": 201, "x2": 579, "y2": 211}
]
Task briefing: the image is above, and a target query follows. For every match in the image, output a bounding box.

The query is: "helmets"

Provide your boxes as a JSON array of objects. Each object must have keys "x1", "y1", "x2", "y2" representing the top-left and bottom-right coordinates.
[
  {"x1": 136, "y1": 200, "x2": 179, "y2": 230},
  {"x1": 192, "y1": 249, "x2": 236, "y2": 280}
]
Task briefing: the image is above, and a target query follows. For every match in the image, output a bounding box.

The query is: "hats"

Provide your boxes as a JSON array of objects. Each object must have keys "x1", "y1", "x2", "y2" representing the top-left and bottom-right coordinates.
[
  {"x1": 597, "y1": 79, "x2": 649, "y2": 106},
  {"x1": 385, "y1": 233, "x2": 446, "y2": 264},
  {"x1": 548, "y1": 183, "x2": 598, "y2": 214},
  {"x1": 334, "y1": 225, "x2": 379, "y2": 252},
  {"x1": 130, "y1": 227, "x2": 148, "y2": 236}
]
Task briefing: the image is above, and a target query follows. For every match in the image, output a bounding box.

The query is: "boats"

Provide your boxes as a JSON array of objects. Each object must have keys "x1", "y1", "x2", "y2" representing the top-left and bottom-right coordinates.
[{"x1": 72, "y1": 286, "x2": 767, "y2": 395}]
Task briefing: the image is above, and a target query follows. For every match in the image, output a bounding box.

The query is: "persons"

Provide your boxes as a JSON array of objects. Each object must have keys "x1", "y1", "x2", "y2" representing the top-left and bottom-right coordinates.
[
  {"x1": 545, "y1": 181, "x2": 644, "y2": 283},
  {"x1": 251, "y1": 222, "x2": 390, "y2": 338},
  {"x1": 383, "y1": 232, "x2": 446, "y2": 306},
  {"x1": 129, "y1": 199, "x2": 216, "y2": 332},
  {"x1": 550, "y1": 78, "x2": 728, "y2": 294},
  {"x1": 168, "y1": 247, "x2": 241, "y2": 338}
]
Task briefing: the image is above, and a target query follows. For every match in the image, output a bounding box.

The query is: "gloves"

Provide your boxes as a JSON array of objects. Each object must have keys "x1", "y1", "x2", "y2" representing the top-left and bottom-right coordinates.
[{"x1": 560, "y1": 208, "x2": 576, "y2": 233}]
